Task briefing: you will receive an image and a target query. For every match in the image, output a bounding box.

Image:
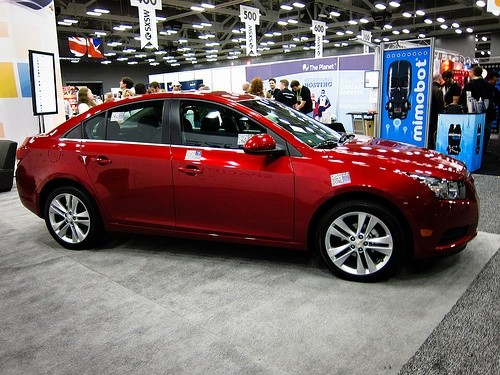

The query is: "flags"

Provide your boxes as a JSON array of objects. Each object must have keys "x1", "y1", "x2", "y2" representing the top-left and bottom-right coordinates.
[{"x1": 68, "y1": 36, "x2": 104, "y2": 59}]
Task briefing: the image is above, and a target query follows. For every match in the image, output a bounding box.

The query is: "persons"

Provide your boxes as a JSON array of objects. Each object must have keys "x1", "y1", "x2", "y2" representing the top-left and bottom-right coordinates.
[
  {"x1": 427, "y1": 62, "x2": 499, "y2": 159},
  {"x1": 63, "y1": 75, "x2": 315, "y2": 133},
  {"x1": 318, "y1": 90, "x2": 332, "y2": 119}
]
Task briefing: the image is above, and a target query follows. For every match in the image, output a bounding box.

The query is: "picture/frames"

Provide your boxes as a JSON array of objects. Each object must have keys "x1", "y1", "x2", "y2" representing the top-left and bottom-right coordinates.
[{"x1": 28, "y1": 49, "x2": 58, "y2": 116}]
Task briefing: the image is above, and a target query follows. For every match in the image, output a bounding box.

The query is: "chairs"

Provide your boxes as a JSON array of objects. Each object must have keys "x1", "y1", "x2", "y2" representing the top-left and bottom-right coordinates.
[
  {"x1": 184, "y1": 114, "x2": 238, "y2": 147},
  {"x1": 138, "y1": 116, "x2": 159, "y2": 142},
  {"x1": 97, "y1": 121, "x2": 131, "y2": 140}
]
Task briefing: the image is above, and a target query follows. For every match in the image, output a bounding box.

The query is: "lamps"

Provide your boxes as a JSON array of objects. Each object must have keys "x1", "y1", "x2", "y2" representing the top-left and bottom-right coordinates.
[
  {"x1": 282, "y1": 43, "x2": 297, "y2": 53},
  {"x1": 302, "y1": 44, "x2": 315, "y2": 50},
  {"x1": 293, "y1": 35, "x2": 310, "y2": 42},
  {"x1": 402, "y1": 8, "x2": 463, "y2": 37},
  {"x1": 373, "y1": 0, "x2": 401, "y2": 11},
  {"x1": 330, "y1": 12, "x2": 341, "y2": 17},
  {"x1": 335, "y1": 29, "x2": 354, "y2": 35},
  {"x1": 348, "y1": 17, "x2": 369, "y2": 26},
  {"x1": 382, "y1": 22, "x2": 400, "y2": 35},
  {"x1": 277, "y1": 2, "x2": 305, "y2": 26},
  {"x1": 227, "y1": 27, "x2": 281, "y2": 60},
  {"x1": 374, "y1": 36, "x2": 390, "y2": 43},
  {"x1": 57, "y1": 3, "x2": 220, "y2": 67},
  {"x1": 322, "y1": 39, "x2": 348, "y2": 49}
]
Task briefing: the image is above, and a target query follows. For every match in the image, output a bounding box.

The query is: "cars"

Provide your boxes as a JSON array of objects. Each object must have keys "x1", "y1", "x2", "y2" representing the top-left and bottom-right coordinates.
[{"x1": 15, "y1": 91, "x2": 480, "y2": 283}]
